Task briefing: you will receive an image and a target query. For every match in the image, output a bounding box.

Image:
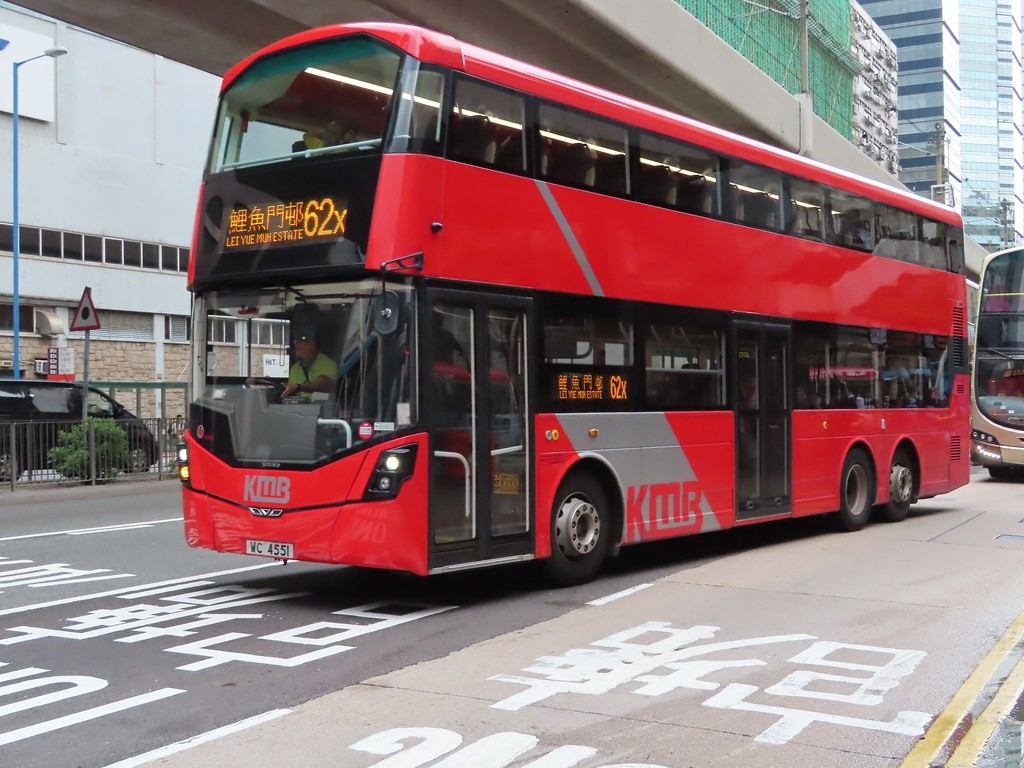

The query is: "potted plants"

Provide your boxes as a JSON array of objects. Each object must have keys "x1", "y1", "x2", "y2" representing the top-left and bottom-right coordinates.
[{"x1": 46, "y1": 415, "x2": 131, "y2": 486}]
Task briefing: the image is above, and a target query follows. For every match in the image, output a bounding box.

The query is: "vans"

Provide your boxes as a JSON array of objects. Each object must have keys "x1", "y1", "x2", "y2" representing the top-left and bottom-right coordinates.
[{"x1": 0, "y1": 376, "x2": 160, "y2": 482}]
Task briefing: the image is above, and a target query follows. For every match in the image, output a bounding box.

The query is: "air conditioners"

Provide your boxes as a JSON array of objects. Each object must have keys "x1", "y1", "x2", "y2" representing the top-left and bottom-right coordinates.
[{"x1": 34, "y1": 359, "x2": 50, "y2": 376}]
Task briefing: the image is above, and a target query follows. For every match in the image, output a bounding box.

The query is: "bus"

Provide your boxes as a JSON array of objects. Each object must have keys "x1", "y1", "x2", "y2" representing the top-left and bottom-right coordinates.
[
  {"x1": 965, "y1": 246, "x2": 1024, "y2": 481},
  {"x1": 176, "y1": 21, "x2": 973, "y2": 588}
]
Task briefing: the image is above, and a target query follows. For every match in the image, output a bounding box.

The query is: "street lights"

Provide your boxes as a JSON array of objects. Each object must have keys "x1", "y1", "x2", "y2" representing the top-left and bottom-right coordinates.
[{"x1": 12, "y1": 45, "x2": 69, "y2": 379}]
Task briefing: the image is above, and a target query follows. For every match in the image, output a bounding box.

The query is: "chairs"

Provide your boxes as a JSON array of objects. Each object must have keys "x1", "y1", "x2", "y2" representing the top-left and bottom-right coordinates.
[{"x1": 447, "y1": 110, "x2": 946, "y2": 274}]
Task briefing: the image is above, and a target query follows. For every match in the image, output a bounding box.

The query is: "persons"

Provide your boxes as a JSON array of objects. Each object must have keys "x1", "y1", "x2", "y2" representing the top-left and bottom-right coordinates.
[
  {"x1": 303, "y1": 120, "x2": 350, "y2": 149},
  {"x1": 168, "y1": 415, "x2": 184, "y2": 449},
  {"x1": 835, "y1": 210, "x2": 864, "y2": 245},
  {"x1": 791, "y1": 374, "x2": 874, "y2": 408},
  {"x1": 280, "y1": 327, "x2": 340, "y2": 404}
]
